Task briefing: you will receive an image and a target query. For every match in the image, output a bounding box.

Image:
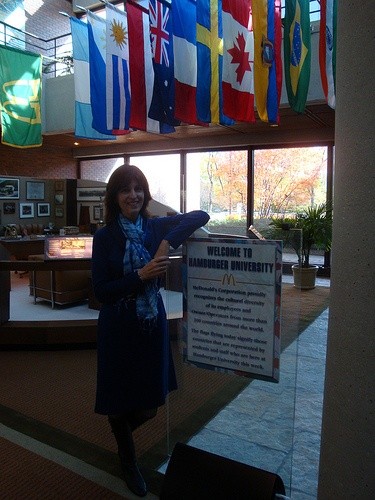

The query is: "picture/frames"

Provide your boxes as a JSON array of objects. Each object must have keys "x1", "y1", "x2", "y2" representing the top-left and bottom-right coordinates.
[
  {"x1": 37, "y1": 202, "x2": 51, "y2": 217},
  {"x1": 26, "y1": 181, "x2": 45, "y2": 200},
  {"x1": 19, "y1": 202, "x2": 35, "y2": 219},
  {"x1": 54, "y1": 194, "x2": 64, "y2": 204},
  {"x1": 55, "y1": 181, "x2": 63, "y2": 191},
  {"x1": 3, "y1": 203, "x2": 15, "y2": 214},
  {"x1": 0, "y1": 177, "x2": 20, "y2": 200}
]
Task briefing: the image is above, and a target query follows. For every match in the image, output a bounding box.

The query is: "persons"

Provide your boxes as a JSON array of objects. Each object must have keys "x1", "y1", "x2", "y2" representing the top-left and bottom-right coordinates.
[{"x1": 90, "y1": 162, "x2": 210, "y2": 496}]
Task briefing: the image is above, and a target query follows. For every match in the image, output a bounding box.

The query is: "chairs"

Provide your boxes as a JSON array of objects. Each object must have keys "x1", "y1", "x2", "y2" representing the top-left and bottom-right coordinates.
[{"x1": 27, "y1": 224, "x2": 43, "y2": 234}]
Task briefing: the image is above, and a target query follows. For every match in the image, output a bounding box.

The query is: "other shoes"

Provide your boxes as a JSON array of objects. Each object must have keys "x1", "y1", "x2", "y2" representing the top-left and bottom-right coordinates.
[{"x1": 120, "y1": 460, "x2": 147, "y2": 497}]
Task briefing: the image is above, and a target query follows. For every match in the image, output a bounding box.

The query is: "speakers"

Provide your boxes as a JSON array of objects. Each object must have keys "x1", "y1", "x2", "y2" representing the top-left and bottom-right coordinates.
[{"x1": 158, "y1": 442, "x2": 285, "y2": 499}]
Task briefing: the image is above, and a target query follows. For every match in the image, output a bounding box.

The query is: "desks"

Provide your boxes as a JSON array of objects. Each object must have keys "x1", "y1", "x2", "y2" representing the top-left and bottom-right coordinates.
[
  {"x1": 29, "y1": 255, "x2": 93, "y2": 309},
  {"x1": 0, "y1": 234, "x2": 94, "y2": 274}
]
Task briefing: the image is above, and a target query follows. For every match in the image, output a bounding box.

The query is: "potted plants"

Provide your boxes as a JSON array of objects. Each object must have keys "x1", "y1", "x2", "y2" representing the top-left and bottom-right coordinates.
[{"x1": 260, "y1": 200, "x2": 333, "y2": 287}]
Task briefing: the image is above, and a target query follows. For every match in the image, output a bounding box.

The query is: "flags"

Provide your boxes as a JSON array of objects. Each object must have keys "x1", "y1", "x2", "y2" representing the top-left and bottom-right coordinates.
[
  {"x1": 69, "y1": 0, "x2": 339, "y2": 144},
  {"x1": 0, "y1": 45, "x2": 42, "y2": 148}
]
